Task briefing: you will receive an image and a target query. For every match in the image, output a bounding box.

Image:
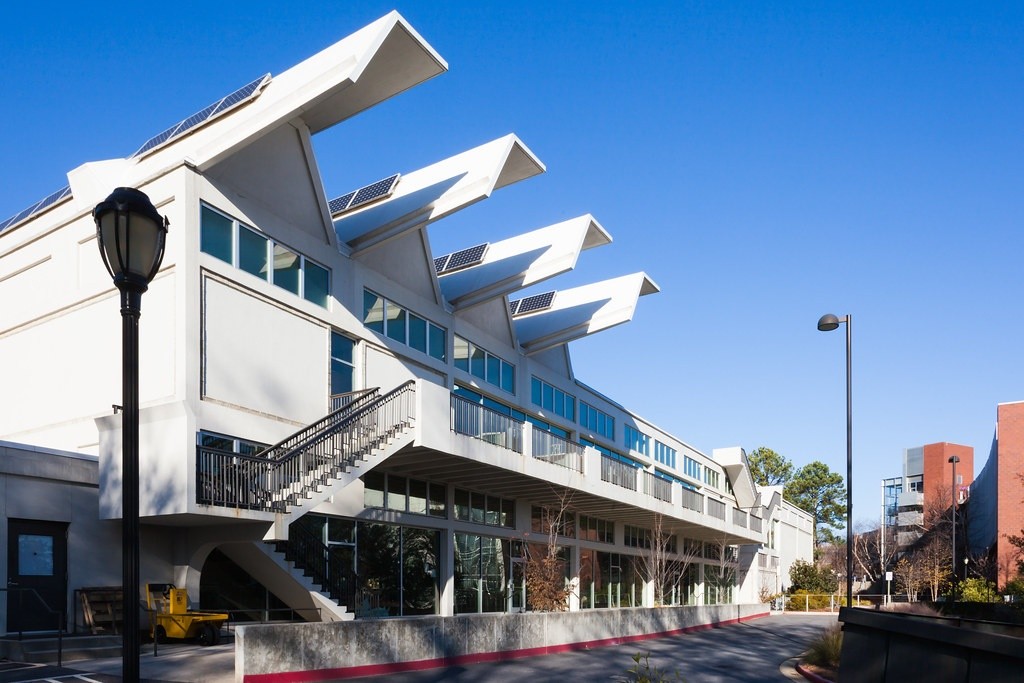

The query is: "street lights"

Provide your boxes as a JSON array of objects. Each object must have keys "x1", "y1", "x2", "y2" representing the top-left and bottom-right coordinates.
[
  {"x1": 946, "y1": 455, "x2": 962, "y2": 579},
  {"x1": 963, "y1": 557, "x2": 969, "y2": 581},
  {"x1": 837, "y1": 573, "x2": 843, "y2": 595},
  {"x1": 816, "y1": 313, "x2": 854, "y2": 607},
  {"x1": 91, "y1": 185, "x2": 170, "y2": 683}
]
